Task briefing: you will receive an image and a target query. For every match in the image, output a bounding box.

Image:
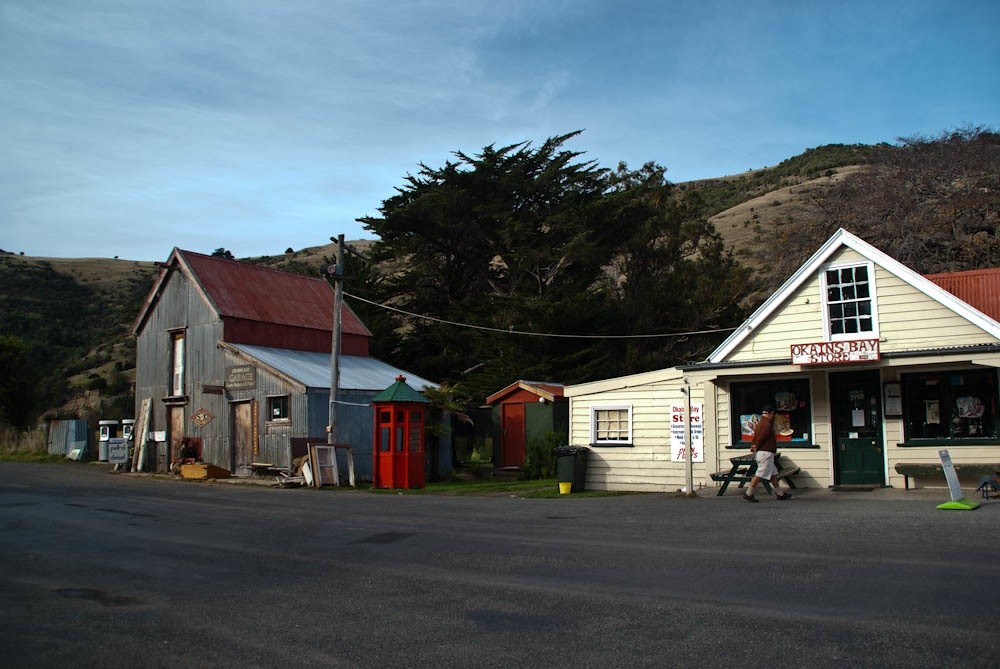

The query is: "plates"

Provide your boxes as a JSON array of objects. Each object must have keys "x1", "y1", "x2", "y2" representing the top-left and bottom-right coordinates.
[{"x1": 780, "y1": 429, "x2": 794, "y2": 436}]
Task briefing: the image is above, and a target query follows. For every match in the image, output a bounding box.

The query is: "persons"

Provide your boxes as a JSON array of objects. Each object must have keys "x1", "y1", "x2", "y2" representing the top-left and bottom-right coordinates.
[{"x1": 743, "y1": 406, "x2": 793, "y2": 502}]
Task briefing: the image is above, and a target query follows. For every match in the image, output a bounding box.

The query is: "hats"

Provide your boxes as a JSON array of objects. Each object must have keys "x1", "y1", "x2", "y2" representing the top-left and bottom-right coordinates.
[{"x1": 762, "y1": 405, "x2": 778, "y2": 414}]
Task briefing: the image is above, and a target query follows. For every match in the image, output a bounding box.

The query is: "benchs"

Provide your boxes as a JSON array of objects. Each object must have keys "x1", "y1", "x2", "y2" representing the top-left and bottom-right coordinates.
[
  {"x1": 895, "y1": 463, "x2": 1000, "y2": 490},
  {"x1": 709, "y1": 467, "x2": 746, "y2": 478},
  {"x1": 776, "y1": 467, "x2": 799, "y2": 478}
]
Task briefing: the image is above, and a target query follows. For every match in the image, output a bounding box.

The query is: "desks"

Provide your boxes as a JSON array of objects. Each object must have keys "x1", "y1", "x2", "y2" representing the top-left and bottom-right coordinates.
[{"x1": 717, "y1": 453, "x2": 797, "y2": 498}]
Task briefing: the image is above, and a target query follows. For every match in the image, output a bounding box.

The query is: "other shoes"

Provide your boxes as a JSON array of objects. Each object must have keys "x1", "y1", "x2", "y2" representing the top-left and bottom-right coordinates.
[
  {"x1": 777, "y1": 493, "x2": 793, "y2": 500},
  {"x1": 743, "y1": 491, "x2": 759, "y2": 503}
]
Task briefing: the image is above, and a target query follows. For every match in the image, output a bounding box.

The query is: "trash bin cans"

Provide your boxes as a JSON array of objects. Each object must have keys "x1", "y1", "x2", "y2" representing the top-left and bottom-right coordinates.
[{"x1": 553, "y1": 446, "x2": 589, "y2": 493}]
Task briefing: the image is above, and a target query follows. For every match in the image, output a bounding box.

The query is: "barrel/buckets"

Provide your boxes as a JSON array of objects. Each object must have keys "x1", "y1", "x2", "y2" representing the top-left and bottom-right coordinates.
[{"x1": 559, "y1": 482, "x2": 571, "y2": 495}]
